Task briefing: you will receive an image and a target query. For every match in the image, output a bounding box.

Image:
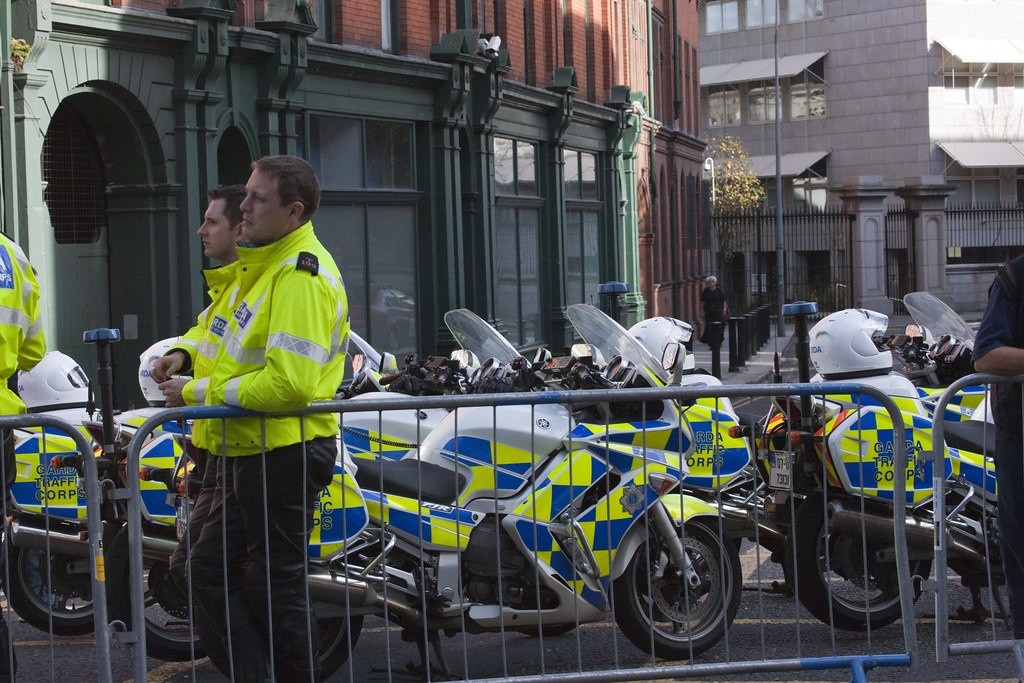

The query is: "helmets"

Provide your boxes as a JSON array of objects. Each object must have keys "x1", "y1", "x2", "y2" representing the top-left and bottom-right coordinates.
[
  {"x1": 619, "y1": 316, "x2": 694, "y2": 376},
  {"x1": 138, "y1": 335, "x2": 195, "y2": 408},
  {"x1": 17, "y1": 350, "x2": 92, "y2": 426},
  {"x1": 807, "y1": 308, "x2": 894, "y2": 380}
]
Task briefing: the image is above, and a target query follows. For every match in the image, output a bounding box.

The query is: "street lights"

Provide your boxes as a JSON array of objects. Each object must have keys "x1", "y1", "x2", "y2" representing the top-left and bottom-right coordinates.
[{"x1": 704, "y1": 157, "x2": 716, "y2": 276}]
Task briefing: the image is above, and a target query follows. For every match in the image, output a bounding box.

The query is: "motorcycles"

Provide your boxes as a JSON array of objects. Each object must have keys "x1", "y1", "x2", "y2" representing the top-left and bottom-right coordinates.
[
  {"x1": 729, "y1": 292, "x2": 1010, "y2": 658},
  {"x1": 0, "y1": 303, "x2": 759, "y2": 683}
]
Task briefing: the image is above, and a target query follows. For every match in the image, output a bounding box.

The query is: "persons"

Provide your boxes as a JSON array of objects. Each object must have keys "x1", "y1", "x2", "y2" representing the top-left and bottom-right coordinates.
[
  {"x1": 0, "y1": 230, "x2": 45, "y2": 683},
  {"x1": 701, "y1": 275, "x2": 732, "y2": 350},
  {"x1": 969, "y1": 253, "x2": 1022, "y2": 640},
  {"x1": 148, "y1": 153, "x2": 352, "y2": 683}
]
